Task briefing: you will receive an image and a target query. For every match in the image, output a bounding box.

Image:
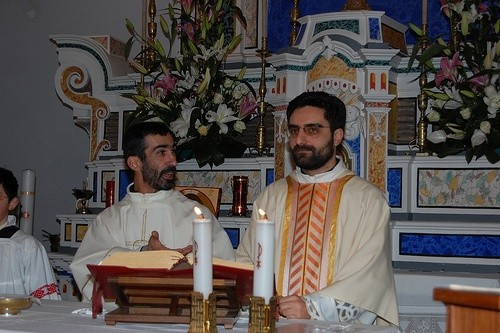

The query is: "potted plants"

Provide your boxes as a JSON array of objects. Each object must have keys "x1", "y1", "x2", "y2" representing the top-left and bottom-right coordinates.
[{"x1": 41, "y1": 230, "x2": 61, "y2": 252}]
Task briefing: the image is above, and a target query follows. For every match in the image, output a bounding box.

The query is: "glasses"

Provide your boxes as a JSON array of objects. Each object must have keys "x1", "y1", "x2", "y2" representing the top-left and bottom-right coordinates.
[
  {"x1": 287, "y1": 123, "x2": 337, "y2": 138},
  {"x1": 0, "y1": 194, "x2": 10, "y2": 200}
]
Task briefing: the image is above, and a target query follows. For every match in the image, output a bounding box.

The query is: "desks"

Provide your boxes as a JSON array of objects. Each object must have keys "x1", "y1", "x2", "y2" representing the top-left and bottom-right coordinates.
[{"x1": 0, "y1": 300, "x2": 403, "y2": 333}]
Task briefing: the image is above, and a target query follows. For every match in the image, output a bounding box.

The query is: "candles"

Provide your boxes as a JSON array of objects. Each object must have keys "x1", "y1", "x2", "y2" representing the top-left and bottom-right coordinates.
[
  {"x1": 20, "y1": 170, "x2": 35, "y2": 235},
  {"x1": 193, "y1": 207, "x2": 212, "y2": 299},
  {"x1": 262, "y1": 0, "x2": 267, "y2": 37},
  {"x1": 142, "y1": 0, "x2": 147, "y2": 35},
  {"x1": 422, "y1": 0, "x2": 427, "y2": 24},
  {"x1": 253, "y1": 209, "x2": 273, "y2": 305}
]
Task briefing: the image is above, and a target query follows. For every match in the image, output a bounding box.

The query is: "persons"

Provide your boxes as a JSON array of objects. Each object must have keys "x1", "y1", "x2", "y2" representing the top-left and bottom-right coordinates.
[
  {"x1": 68, "y1": 122, "x2": 236, "y2": 306},
  {"x1": 233, "y1": 90, "x2": 400, "y2": 332},
  {"x1": 0, "y1": 167, "x2": 64, "y2": 304}
]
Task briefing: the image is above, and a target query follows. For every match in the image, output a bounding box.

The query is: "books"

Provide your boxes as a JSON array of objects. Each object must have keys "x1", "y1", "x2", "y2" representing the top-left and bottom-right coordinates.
[{"x1": 86, "y1": 250, "x2": 278, "y2": 301}]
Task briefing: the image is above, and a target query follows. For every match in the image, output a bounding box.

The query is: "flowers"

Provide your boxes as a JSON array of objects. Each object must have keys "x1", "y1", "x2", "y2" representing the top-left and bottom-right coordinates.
[
  {"x1": 406, "y1": 0, "x2": 500, "y2": 164},
  {"x1": 121, "y1": 0, "x2": 266, "y2": 171}
]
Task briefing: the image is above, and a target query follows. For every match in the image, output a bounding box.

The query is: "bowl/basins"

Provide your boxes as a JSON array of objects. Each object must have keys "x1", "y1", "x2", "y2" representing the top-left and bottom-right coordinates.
[{"x1": 0, "y1": 293, "x2": 32, "y2": 316}]
